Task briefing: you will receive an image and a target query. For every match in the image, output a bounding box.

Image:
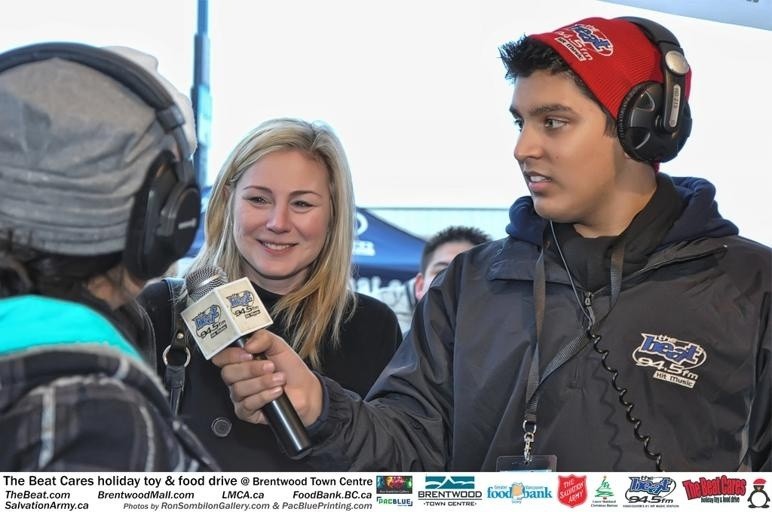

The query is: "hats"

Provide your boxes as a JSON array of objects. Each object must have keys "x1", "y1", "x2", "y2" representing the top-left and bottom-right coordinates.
[{"x1": 526, "y1": 17, "x2": 692, "y2": 175}]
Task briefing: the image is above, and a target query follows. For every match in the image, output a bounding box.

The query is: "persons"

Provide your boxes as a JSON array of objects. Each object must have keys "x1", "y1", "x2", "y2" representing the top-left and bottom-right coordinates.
[
  {"x1": 211, "y1": 17, "x2": 771, "y2": 471},
  {"x1": 139, "y1": 119, "x2": 404, "y2": 471},
  {"x1": 0, "y1": 43, "x2": 223, "y2": 471},
  {"x1": 415, "y1": 227, "x2": 492, "y2": 301}
]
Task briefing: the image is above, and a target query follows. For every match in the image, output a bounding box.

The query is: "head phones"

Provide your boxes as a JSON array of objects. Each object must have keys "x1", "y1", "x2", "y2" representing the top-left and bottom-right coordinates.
[{"x1": 615, "y1": 16, "x2": 692, "y2": 162}]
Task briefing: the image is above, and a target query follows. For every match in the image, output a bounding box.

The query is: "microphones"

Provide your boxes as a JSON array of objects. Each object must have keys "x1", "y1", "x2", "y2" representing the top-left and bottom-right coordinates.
[{"x1": 180, "y1": 263, "x2": 313, "y2": 460}]
